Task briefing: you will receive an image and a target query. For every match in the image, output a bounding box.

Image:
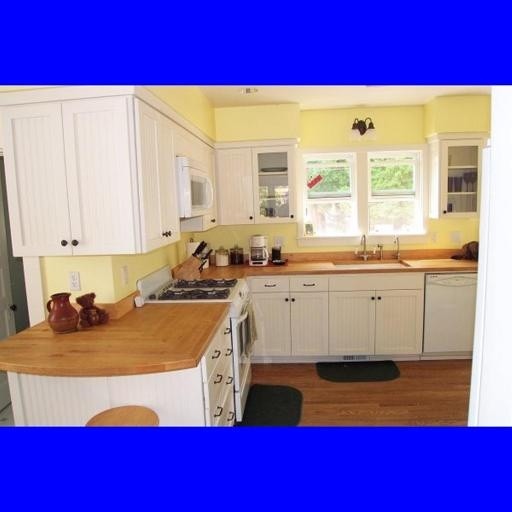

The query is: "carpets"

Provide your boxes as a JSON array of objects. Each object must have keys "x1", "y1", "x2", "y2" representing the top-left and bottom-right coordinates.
[
  {"x1": 315, "y1": 359, "x2": 401, "y2": 382},
  {"x1": 237, "y1": 383, "x2": 304, "y2": 426}
]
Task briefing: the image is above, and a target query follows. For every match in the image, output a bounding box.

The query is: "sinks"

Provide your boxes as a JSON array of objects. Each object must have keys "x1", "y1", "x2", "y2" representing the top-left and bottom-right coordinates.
[{"x1": 328, "y1": 259, "x2": 413, "y2": 270}]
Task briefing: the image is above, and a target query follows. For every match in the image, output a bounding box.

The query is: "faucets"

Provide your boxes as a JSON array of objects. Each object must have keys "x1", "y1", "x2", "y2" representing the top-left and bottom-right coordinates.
[
  {"x1": 392, "y1": 236, "x2": 402, "y2": 259},
  {"x1": 359, "y1": 232, "x2": 372, "y2": 259},
  {"x1": 377, "y1": 242, "x2": 384, "y2": 260}
]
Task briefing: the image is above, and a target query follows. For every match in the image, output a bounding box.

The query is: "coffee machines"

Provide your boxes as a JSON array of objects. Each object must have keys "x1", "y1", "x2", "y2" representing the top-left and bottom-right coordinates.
[{"x1": 248, "y1": 235, "x2": 269, "y2": 266}]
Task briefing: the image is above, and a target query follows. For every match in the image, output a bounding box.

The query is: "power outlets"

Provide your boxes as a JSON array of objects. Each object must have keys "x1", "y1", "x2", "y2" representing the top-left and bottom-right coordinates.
[{"x1": 69, "y1": 272, "x2": 80, "y2": 292}]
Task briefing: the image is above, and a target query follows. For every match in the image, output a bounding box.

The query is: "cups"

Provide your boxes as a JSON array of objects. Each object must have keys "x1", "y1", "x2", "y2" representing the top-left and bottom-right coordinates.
[
  {"x1": 448, "y1": 203, "x2": 452, "y2": 212},
  {"x1": 265, "y1": 207, "x2": 273, "y2": 216},
  {"x1": 134, "y1": 296, "x2": 145, "y2": 307},
  {"x1": 454, "y1": 176, "x2": 461, "y2": 191},
  {"x1": 448, "y1": 176, "x2": 453, "y2": 192}
]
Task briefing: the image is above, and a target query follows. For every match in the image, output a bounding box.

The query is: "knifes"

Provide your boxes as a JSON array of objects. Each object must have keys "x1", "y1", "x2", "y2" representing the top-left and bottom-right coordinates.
[{"x1": 192, "y1": 240, "x2": 213, "y2": 270}]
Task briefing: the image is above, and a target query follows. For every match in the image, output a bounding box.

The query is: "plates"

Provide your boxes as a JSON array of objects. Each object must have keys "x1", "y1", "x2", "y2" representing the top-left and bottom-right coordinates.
[
  {"x1": 262, "y1": 167, "x2": 288, "y2": 172},
  {"x1": 272, "y1": 259, "x2": 286, "y2": 265}
]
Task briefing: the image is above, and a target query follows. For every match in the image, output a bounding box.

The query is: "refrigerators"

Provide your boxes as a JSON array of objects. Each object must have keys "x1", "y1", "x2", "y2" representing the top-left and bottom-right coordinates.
[{"x1": 423, "y1": 272, "x2": 478, "y2": 358}]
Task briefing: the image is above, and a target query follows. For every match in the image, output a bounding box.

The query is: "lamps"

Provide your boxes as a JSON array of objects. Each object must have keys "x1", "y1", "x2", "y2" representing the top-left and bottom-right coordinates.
[{"x1": 350, "y1": 117, "x2": 378, "y2": 142}]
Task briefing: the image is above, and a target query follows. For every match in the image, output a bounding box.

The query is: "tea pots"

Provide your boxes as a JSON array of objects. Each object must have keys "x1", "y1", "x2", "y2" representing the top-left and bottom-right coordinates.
[{"x1": 45, "y1": 293, "x2": 79, "y2": 336}]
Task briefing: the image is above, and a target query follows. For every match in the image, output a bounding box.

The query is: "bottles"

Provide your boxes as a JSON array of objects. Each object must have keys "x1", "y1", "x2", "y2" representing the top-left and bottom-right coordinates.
[
  {"x1": 271, "y1": 240, "x2": 282, "y2": 259},
  {"x1": 215, "y1": 245, "x2": 244, "y2": 267}
]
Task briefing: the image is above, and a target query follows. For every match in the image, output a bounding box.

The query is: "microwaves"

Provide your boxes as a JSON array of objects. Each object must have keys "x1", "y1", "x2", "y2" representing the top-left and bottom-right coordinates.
[{"x1": 176, "y1": 155, "x2": 214, "y2": 221}]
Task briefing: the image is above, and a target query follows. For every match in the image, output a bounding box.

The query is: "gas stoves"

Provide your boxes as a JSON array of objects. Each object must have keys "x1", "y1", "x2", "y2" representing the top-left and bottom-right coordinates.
[{"x1": 149, "y1": 278, "x2": 238, "y2": 299}]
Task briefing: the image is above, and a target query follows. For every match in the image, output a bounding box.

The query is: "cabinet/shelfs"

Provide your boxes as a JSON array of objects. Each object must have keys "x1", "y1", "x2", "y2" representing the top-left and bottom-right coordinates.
[
  {"x1": 0, "y1": 84, "x2": 136, "y2": 258},
  {"x1": 328, "y1": 272, "x2": 424, "y2": 364},
  {"x1": 204, "y1": 316, "x2": 235, "y2": 427},
  {"x1": 211, "y1": 147, "x2": 296, "y2": 226},
  {"x1": 137, "y1": 97, "x2": 181, "y2": 254},
  {"x1": 247, "y1": 276, "x2": 328, "y2": 365},
  {"x1": 428, "y1": 134, "x2": 484, "y2": 221}
]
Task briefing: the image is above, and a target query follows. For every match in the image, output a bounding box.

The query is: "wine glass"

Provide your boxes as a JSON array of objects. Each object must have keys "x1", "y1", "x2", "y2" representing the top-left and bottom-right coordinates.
[{"x1": 463, "y1": 172, "x2": 476, "y2": 192}]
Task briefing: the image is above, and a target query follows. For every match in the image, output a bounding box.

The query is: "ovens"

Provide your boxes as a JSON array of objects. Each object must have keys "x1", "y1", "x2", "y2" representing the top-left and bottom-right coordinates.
[{"x1": 230, "y1": 297, "x2": 253, "y2": 423}]
